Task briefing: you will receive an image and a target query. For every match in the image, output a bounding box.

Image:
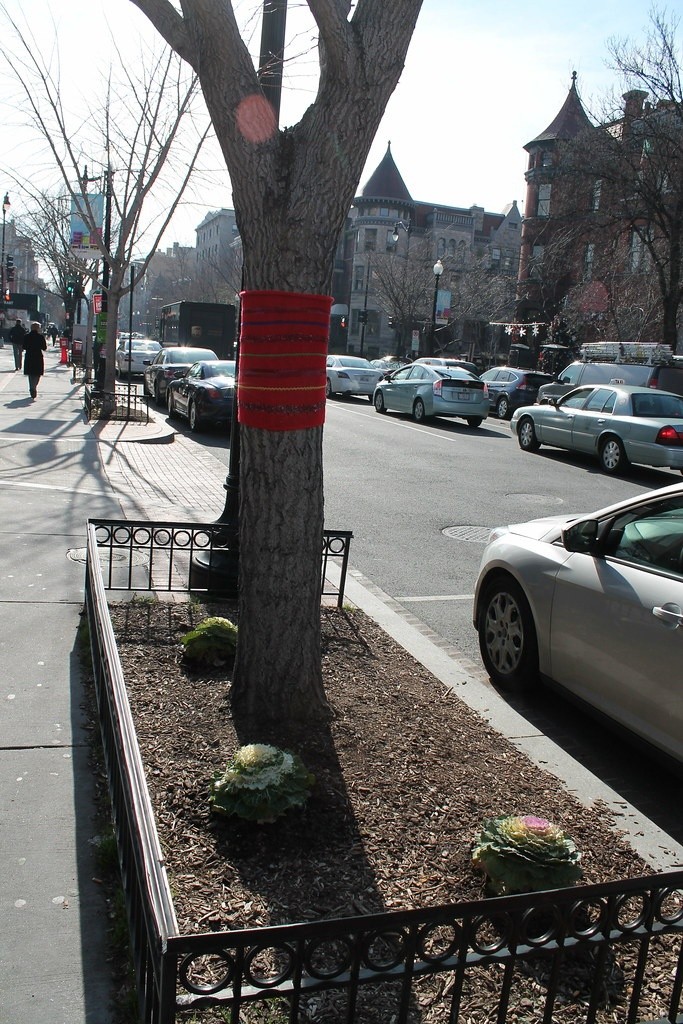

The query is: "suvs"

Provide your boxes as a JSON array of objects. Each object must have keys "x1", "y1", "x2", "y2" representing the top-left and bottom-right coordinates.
[{"x1": 534, "y1": 342, "x2": 683, "y2": 410}]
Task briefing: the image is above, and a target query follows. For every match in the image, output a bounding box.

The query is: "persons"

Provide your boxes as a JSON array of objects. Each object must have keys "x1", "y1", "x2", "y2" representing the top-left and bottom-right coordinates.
[
  {"x1": 50, "y1": 325, "x2": 58, "y2": 346},
  {"x1": 8, "y1": 320, "x2": 26, "y2": 371},
  {"x1": 22, "y1": 322, "x2": 47, "y2": 398}
]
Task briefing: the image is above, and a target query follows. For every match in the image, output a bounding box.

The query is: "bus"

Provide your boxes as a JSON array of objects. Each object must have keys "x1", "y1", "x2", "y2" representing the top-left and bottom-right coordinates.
[{"x1": 155, "y1": 300, "x2": 237, "y2": 360}]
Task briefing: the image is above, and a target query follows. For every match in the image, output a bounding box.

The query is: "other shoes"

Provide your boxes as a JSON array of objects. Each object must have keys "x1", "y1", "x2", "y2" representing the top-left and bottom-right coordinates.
[
  {"x1": 16, "y1": 368, "x2": 19, "y2": 371},
  {"x1": 32, "y1": 397, "x2": 36, "y2": 401}
]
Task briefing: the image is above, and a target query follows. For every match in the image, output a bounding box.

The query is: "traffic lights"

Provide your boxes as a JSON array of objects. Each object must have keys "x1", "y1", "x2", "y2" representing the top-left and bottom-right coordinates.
[
  {"x1": 7, "y1": 255, "x2": 14, "y2": 282},
  {"x1": 358, "y1": 310, "x2": 367, "y2": 323},
  {"x1": 5, "y1": 288, "x2": 10, "y2": 302},
  {"x1": 340, "y1": 317, "x2": 346, "y2": 328},
  {"x1": 67, "y1": 281, "x2": 75, "y2": 296}
]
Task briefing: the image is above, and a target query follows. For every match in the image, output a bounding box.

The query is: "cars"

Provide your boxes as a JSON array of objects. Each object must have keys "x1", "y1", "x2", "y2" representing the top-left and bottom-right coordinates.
[
  {"x1": 510, "y1": 378, "x2": 683, "y2": 479},
  {"x1": 410, "y1": 358, "x2": 481, "y2": 378},
  {"x1": 165, "y1": 360, "x2": 237, "y2": 436},
  {"x1": 370, "y1": 356, "x2": 414, "y2": 377},
  {"x1": 116, "y1": 331, "x2": 146, "y2": 342},
  {"x1": 324, "y1": 354, "x2": 385, "y2": 401},
  {"x1": 477, "y1": 366, "x2": 558, "y2": 420},
  {"x1": 115, "y1": 340, "x2": 164, "y2": 379},
  {"x1": 470, "y1": 479, "x2": 683, "y2": 765},
  {"x1": 372, "y1": 364, "x2": 491, "y2": 428},
  {"x1": 141, "y1": 346, "x2": 221, "y2": 406}
]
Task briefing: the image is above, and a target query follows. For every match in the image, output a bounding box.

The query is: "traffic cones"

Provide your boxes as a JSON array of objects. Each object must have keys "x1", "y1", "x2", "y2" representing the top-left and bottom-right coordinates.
[{"x1": 57, "y1": 347, "x2": 68, "y2": 364}]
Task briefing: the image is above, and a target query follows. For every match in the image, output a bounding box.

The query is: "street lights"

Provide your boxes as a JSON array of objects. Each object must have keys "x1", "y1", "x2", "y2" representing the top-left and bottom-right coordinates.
[
  {"x1": 0, "y1": 190, "x2": 12, "y2": 309},
  {"x1": 389, "y1": 217, "x2": 412, "y2": 357},
  {"x1": 99, "y1": 154, "x2": 115, "y2": 383},
  {"x1": 428, "y1": 258, "x2": 445, "y2": 357}
]
